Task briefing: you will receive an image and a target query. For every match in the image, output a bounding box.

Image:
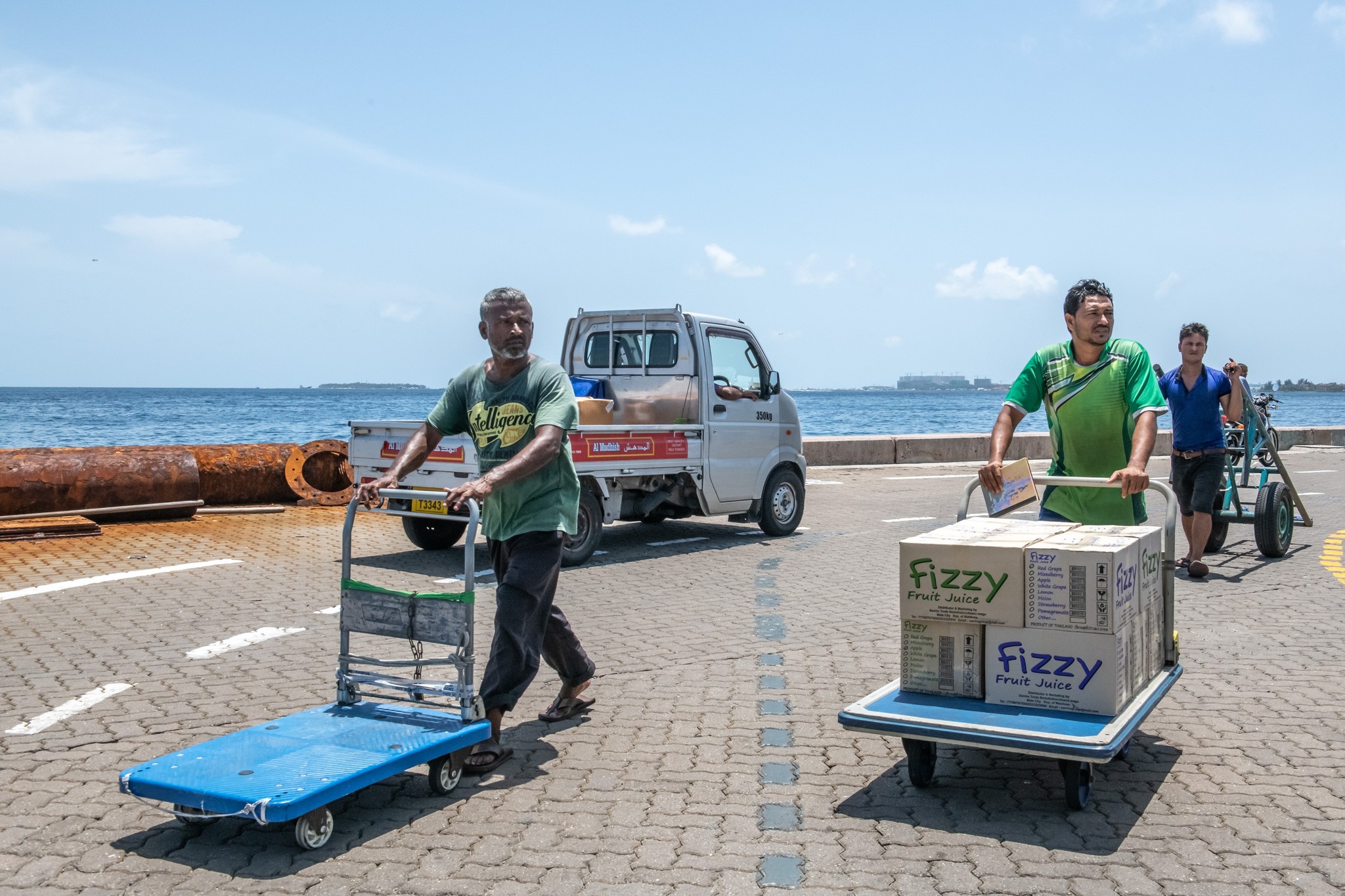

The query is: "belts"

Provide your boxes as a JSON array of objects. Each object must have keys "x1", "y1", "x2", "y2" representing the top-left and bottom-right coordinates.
[{"x1": 1172, "y1": 447, "x2": 1226, "y2": 460}]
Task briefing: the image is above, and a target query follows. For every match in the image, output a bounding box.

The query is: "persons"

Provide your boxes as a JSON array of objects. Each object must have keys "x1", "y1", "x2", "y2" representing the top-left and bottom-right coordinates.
[
  {"x1": 673, "y1": 343, "x2": 760, "y2": 402},
  {"x1": 613, "y1": 341, "x2": 624, "y2": 366},
  {"x1": 1157, "y1": 322, "x2": 1242, "y2": 578},
  {"x1": 356, "y1": 287, "x2": 596, "y2": 776},
  {"x1": 977, "y1": 279, "x2": 1158, "y2": 524},
  {"x1": 1229, "y1": 364, "x2": 1269, "y2": 448}
]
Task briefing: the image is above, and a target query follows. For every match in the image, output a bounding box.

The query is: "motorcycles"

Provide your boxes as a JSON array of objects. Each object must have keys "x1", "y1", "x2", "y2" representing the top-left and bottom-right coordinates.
[{"x1": 1223, "y1": 382, "x2": 1285, "y2": 466}]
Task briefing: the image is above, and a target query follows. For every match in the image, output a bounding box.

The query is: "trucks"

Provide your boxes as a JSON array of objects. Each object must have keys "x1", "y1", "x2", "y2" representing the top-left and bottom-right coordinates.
[{"x1": 347, "y1": 304, "x2": 806, "y2": 567}]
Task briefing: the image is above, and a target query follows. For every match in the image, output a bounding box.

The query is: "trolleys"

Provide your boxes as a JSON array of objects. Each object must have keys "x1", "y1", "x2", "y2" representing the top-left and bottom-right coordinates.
[
  {"x1": 837, "y1": 475, "x2": 1182, "y2": 813},
  {"x1": 120, "y1": 488, "x2": 491, "y2": 848},
  {"x1": 1154, "y1": 362, "x2": 1312, "y2": 559}
]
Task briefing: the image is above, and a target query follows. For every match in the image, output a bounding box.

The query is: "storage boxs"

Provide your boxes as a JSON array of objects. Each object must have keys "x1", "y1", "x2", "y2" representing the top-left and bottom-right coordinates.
[
  {"x1": 897, "y1": 527, "x2": 1051, "y2": 630},
  {"x1": 1024, "y1": 530, "x2": 1141, "y2": 632},
  {"x1": 1131, "y1": 608, "x2": 1150, "y2": 698},
  {"x1": 574, "y1": 395, "x2": 614, "y2": 426},
  {"x1": 981, "y1": 624, "x2": 1133, "y2": 716},
  {"x1": 900, "y1": 616, "x2": 985, "y2": 700},
  {"x1": 1146, "y1": 595, "x2": 1166, "y2": 680},
  {"x1": 948, "y1": 515, "x2": 1082, "y2": 540},
  {"x1": 1069, "y1": 524, "x2": 1162, "y2": 612}
]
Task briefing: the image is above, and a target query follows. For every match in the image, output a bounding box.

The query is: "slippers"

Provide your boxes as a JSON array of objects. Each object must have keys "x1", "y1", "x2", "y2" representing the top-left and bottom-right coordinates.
[
  {"x1": 538, "y1": 692, "x2": 596, "y2": 721},
  {"x1": 1175, "y1": 557, "x2": 1190, "y2": 567},
  {"x1": 1187, "y1": 560, "x2": 1209, "y2": 577},
  {"x1": 463, "y1": 743, "x2": 514, "y2": 773}
]
